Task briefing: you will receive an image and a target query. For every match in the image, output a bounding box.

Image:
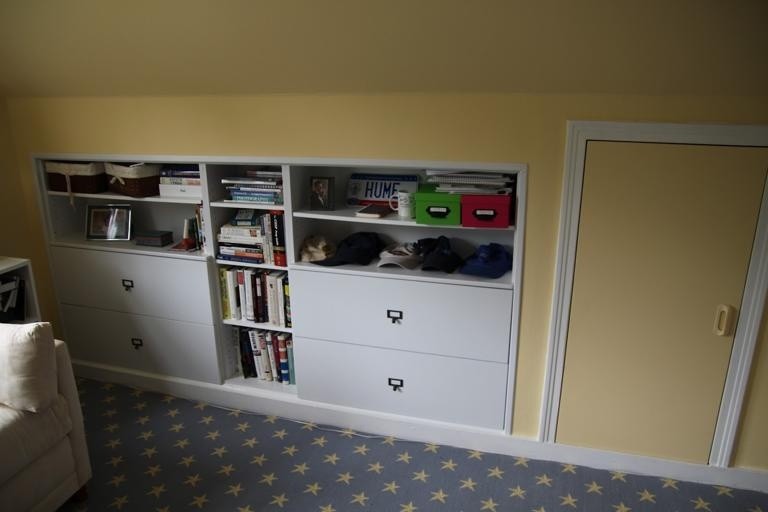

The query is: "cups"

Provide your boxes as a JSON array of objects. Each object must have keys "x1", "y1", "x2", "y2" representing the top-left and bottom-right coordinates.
[{"x1": 388, "y1": 190, "x2": 415, "y2": 218}]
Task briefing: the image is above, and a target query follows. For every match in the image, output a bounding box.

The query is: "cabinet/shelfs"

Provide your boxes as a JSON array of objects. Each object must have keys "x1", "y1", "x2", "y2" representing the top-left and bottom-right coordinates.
[
  {"x1": 203, "y1": 159, "x2": 297, "y2": 420},
  {"x1": 0, "y1": 253, "x2": 42, "y2": 323},
  {"x1": 292, "y1": 160, "x2": 529, "y2": 453},
  {"x1": 32, "y1": 153, "x2": 223, "y2": 408}
]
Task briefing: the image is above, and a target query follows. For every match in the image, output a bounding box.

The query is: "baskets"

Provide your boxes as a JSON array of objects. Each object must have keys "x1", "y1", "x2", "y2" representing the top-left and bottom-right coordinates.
[
  {"x1": 43, "y1": 161, "x2": 106, "y2": 194},
  {"x1": 103, "y1": 160, "x2": 165, "y2": 199}
]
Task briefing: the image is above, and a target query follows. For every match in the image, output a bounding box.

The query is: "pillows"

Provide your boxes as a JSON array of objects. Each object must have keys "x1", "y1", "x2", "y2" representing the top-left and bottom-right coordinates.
[{"x1": 1, "y1": 319, "x2": 57, "y2": 414}]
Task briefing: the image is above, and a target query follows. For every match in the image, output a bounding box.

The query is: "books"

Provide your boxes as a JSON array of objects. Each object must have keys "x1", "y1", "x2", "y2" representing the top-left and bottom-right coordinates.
[
  {"x1": 170, "y1": 205, "x2": 203, "y2": 253},
  {"x1": 214, "y1": 209, "x2": 296, "y2": 387},
  {"x1": 156, "y1": 167, "x2": 203, "y2": 197},
  {"x1": 354, "y1": 203, "x2": 396, "y2": 219},
  {"x1": 216, "y1": 167, "x2": 284, "y2": 204}
]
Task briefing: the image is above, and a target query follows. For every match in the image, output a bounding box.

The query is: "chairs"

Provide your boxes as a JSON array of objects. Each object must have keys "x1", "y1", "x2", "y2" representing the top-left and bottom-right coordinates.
[{"x1": 0, "y1": 322, "x2": 93, "y2": 512}]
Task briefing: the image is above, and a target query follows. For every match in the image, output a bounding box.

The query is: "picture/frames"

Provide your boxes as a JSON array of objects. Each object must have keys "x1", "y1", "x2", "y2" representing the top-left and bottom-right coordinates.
[
  {"x1": 309, "y1": 178, "x2": 334, "y2": 209},
  {"x1": 84, "y1": 202, "x2": 132, "y2": 245}
]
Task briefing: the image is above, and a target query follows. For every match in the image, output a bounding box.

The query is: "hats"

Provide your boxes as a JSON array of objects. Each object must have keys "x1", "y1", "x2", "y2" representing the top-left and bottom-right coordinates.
[
  {"x1": 328, "y1": 231, "x2": 386, "y2": 267},
  {"x1": 375, "y1": 240, "x2": 426, "y2": 272},
  {"x1": 298, "y1": 232, "x2": 346, "y2": 267},
  {"x1": 418, "y1": 235, "x2": 477, "y2": 273}
]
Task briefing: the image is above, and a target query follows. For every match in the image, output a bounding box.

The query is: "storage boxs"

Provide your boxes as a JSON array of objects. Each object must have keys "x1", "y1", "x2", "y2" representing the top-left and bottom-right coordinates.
[
  {"x1": 463, "y1": 190, "x2": 511, "y2": 230},
  {"x1": 412, "y1": 182, "x2": 461, "y2": 227}
]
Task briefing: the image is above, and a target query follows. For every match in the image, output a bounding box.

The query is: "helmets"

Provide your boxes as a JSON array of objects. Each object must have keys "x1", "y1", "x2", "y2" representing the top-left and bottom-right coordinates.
[{"x1": 457, "y1": 241, "x2": 513, "y2": 279}]
